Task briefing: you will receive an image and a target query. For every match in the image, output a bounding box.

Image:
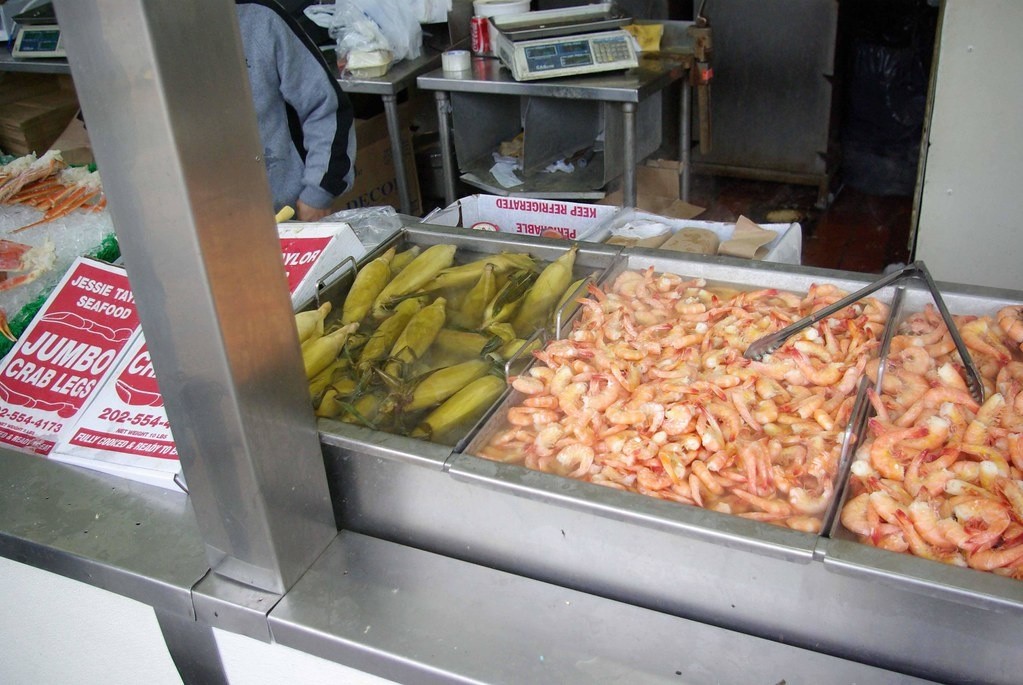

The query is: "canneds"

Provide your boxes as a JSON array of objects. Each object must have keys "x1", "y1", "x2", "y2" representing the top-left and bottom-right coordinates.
[{"x1": 470, "y1": 15, "x2": 490, "y2": 53}]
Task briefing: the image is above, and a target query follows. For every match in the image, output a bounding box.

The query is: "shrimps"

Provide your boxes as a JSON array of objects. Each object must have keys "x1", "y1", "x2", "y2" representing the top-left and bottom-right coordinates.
[{"x1": 475, "y1": 264, "x2": 1023, "y2": 585}]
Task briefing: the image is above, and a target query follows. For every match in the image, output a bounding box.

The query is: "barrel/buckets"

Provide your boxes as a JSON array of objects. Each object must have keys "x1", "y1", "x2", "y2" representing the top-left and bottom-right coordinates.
[{"x1": 472, "y1": 0, "x2": 531, "y2": 57}]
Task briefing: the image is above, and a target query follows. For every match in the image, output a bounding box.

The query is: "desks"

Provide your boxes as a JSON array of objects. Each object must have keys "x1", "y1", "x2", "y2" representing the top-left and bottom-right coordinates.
[
  {"x1": 0, "y1": 35, "x2": 472, "y2": 216},
  {"x1": 417, "y1": 51, "x2": 695, "y2": 209}
]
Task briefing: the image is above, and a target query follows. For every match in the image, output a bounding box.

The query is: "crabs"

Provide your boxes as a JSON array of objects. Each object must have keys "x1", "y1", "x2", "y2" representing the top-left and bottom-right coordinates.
[{"x1": 0, "y1": 148, "x2": 106, "y2": 343}]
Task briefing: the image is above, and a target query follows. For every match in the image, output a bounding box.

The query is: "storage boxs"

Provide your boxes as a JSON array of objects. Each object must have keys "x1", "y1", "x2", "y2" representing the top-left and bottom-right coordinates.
[
  {"x1": 423, "y1": 194, "x2": 620, "y2": 240},
  {"x1": 277, "y1": 220, "x2": 368, "y2": 310},
  {"x1": 596, "y1": 159, "x2": 706, "y2": 219},
  {"x1": 0, "y1": 90, "x2": 79, "y2": 159},
  {"x1": 0, "y1": 255, "x2": 142, "y2": 459},
  {"x1": 48, "y1": 330, "x2": 189, "y2": 494},
  {"x1": 587, "y1": 208, "x2": 802, "y2": 266},
  {"x1": 329, "y1": 100, "x2": 423, "y2": 217}
]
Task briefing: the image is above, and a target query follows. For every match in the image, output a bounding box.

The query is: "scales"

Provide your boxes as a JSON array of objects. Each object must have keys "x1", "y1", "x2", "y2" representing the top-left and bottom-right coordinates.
[
  {"x1": 12, "y1": 2, "x2": 67, "y2": 58},
  {"x1": 487, "y1": 2, "x2": 638, "y2": 81}
]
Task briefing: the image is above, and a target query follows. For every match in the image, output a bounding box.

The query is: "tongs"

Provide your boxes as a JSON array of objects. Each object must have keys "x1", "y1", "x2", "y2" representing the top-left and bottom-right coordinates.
[{"x1": 744, "y1": 258, "x2": 986, "y2": 407}]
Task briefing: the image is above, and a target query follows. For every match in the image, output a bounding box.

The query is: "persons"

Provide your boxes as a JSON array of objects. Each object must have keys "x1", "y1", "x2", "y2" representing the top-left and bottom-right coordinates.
[{"x1": 235, "y1": 0, "x2": 358, "y2": 224}]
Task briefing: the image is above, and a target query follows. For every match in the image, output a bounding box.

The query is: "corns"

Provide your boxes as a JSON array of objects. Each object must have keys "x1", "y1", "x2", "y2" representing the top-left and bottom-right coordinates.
[{"x1": 292, "y1": 242, "x2": 608, "y2": 445}]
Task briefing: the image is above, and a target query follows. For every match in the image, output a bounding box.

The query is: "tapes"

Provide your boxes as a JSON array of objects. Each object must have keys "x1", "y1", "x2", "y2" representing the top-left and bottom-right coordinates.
[{"x1": 442, "y1": 50, "x2": 471, "y2": 71}]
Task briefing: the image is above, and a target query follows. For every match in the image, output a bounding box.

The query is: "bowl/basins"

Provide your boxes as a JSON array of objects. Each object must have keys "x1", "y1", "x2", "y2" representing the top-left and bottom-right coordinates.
[{"x1": 345, "y1": 50, "x2": 394, "y2": 79}]
